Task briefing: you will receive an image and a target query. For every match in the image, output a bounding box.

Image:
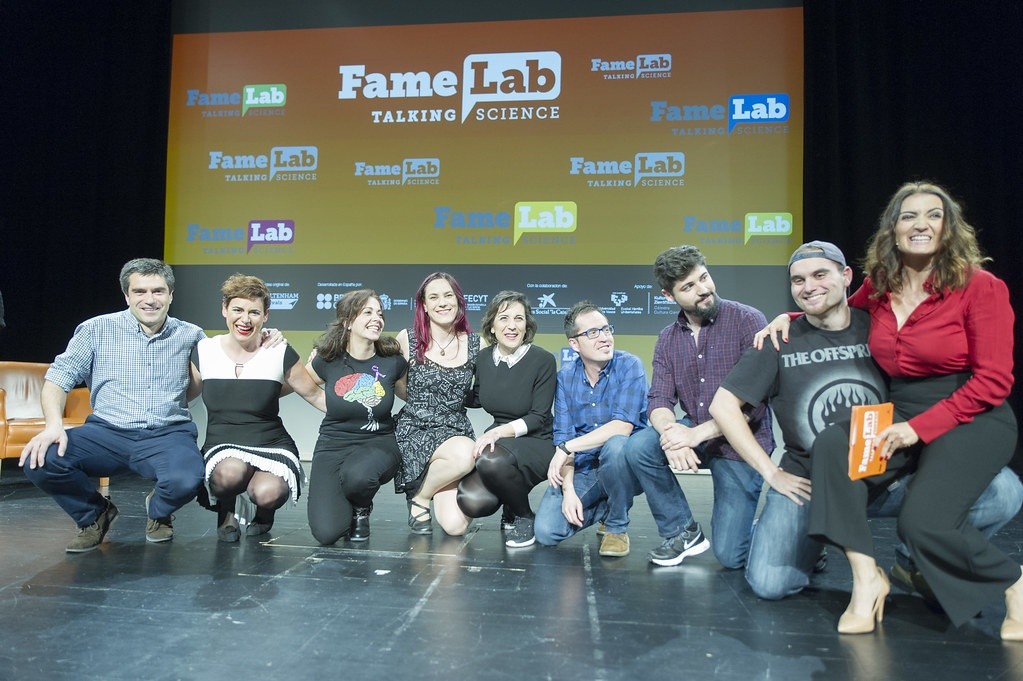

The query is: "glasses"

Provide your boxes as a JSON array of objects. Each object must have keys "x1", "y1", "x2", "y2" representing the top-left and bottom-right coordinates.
[{"x1": 568, "y1": 324, "x2": 614, "y2": 340}]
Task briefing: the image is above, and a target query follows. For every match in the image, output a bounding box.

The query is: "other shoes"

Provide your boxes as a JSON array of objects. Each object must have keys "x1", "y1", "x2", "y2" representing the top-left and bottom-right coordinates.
[
  {"x1": 246, "y1": 507, "x2": 275, "y2": 536},
  {"x1": 217, "y1": 500, "x2": 241, "y2": 542}
]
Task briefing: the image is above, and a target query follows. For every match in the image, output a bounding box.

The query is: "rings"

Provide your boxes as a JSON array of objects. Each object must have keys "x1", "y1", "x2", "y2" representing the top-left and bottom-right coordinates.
[{"x1": 887, "y1": 439, "x2": 893, "y2": 444}]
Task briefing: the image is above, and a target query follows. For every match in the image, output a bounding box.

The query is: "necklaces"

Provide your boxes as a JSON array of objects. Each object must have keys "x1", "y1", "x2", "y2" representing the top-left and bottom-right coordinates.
[{"x1": 432, "y1": 335, "x2": 455, "y2": 356}]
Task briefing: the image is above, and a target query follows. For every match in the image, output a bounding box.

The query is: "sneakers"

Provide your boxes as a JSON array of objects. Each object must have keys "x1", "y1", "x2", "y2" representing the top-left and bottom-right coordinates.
[
  {"x1": 597, "y1": 518, "x2": 606, "y2": 536},
  {"x1": 145, "y1": 488, "x2": 176, "y2": 543},
  {"x1": 501, "y1": 503, "x2": 520, "y2": 529},
  {"x1": 504, "y1": 511, "x2": 535, "y2": 547},
  {"x1": 888, "y1": 561, "x2": 941, "y2": 610},
  {"x1": 814, "y1": 546, "x2": 829, "y2": 572},
  {"x1": 65, "y1": 496, "x2": 119, "y2": 553},
  {"x1": 599, "y1": 531, "x2": 630, "y2": 557},
  {"x1": 646, "y1": 522, "x2": 711, "y2": 566}
]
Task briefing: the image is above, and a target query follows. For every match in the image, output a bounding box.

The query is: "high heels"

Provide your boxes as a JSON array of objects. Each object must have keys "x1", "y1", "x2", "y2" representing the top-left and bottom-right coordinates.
[
  {"x1": 838, "y1": 567, "x2": 890, "y2": 634},
  {"x1": 999, "y1": 564, "x2": 1023, "y2": 640}
]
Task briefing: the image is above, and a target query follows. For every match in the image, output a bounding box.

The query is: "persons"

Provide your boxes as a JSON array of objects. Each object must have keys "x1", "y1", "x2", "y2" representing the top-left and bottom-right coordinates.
[
  {"x1": 534, "y1": 301, "x2": 648, "y2": 557},
  {"x1": 624, "y1": 244, "x2": 776, "y2": 570},
  {"x1": 468, "y1": 290, "x2": 557, "y2": 547},
  {"x1": 753, "y1": 180, "x2": 1023, "y2": 639},
  {"x1": 19, "y1": 259, "x2": 288, "y2": 552},
  {"x1": 280, "y1": 288, "x2": 409, "y2": 545},
  {"x1": 708, "y1": 241, "x2": 1023, "y2": 601},
  {"x1": 308, "y1": 271, "x2": 497, "y2": 536},
  {"x1": 184, "y1": 275, "x2": 326, "y2": 542}
]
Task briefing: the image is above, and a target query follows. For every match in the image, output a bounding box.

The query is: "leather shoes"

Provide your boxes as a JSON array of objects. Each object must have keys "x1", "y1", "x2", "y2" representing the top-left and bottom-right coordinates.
[
  {"x1": 407, "y1": 497, "x2": 433, "y2": 534},
  {"x1": 345, "y1": 504, "x2": 373, "y2": 541}
]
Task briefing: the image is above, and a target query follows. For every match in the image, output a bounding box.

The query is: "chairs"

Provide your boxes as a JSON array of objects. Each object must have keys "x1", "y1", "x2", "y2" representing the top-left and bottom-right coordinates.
[{"x1": 0, "y1": 361, "x2": 111, "y2": 487}]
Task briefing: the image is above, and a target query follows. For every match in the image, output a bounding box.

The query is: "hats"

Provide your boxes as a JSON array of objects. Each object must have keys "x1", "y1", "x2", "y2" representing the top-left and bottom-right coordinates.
[{"x1": 786, "y1": 239, "x2": 850, "y2": 297}]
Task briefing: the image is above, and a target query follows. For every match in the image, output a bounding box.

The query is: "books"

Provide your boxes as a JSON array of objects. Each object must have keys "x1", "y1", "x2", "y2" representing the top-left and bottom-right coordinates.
[{"x1": 848, "y1": 402, "x2": 894, "y2": 480}]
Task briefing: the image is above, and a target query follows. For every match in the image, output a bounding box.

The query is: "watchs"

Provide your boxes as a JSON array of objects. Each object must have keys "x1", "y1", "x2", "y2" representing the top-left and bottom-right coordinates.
[{"x1": 558, "y1": 441, "x2": 571, "y2": 456}]
